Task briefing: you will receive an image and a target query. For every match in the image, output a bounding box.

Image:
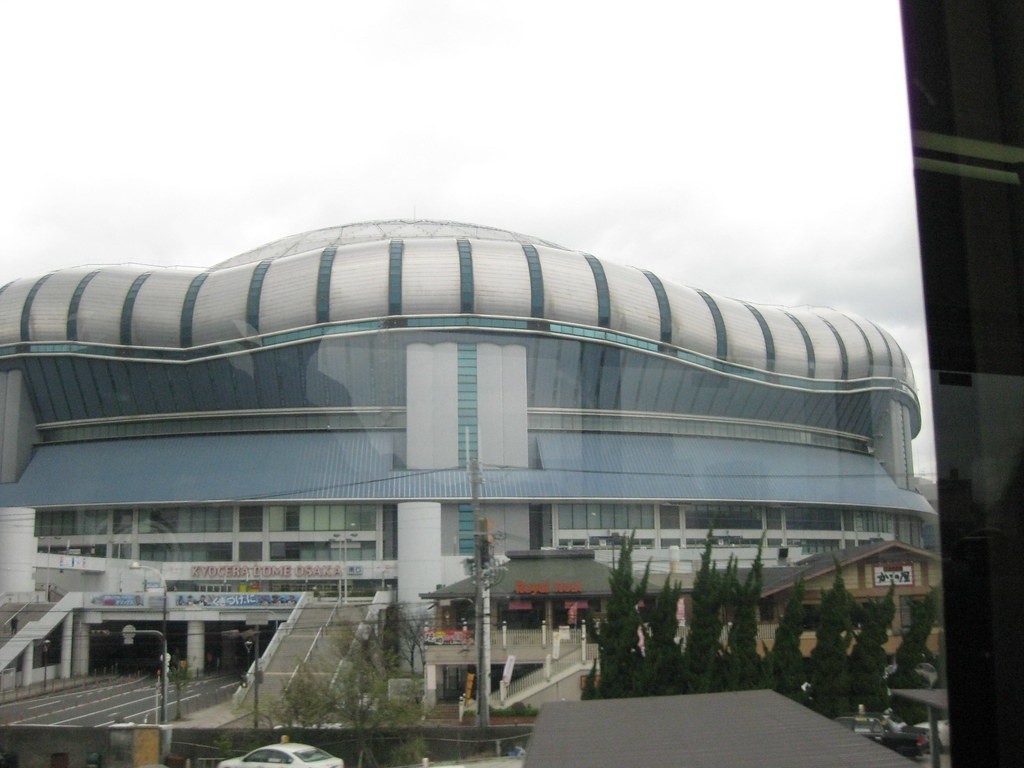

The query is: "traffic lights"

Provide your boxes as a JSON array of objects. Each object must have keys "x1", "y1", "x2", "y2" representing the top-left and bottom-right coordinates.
[{"x1": 88, "y1": 629, "x2": 111, "y2": 636}]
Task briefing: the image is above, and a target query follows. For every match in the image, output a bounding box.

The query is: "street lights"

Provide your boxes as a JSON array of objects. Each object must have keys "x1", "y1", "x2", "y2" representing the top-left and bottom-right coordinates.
[
  {"x1": 130, "y1": 563, "x2": 168, "y2": 725},
  {"x1": 329, "y1": 532, "x2": 358, "y2": 604}
]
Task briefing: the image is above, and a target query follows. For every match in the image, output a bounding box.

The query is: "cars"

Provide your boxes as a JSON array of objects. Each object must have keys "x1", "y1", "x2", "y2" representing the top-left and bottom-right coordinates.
[
  {"x1": 832, "y1": 712, "x2": 930, "y2": 760},
  {"x1": 915, "y1": 718, "x2": 950, "y2": 755},
  {"x1": 217, "y1": 742, "x2": 344, "y2": 768}
]
